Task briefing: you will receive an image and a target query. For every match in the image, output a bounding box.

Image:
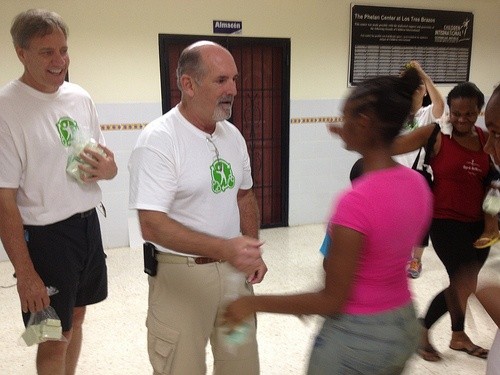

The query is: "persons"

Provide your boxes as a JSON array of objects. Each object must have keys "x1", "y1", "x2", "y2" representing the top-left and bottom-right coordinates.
[
  {"x1": 394, "y1": 59, "x2": 500, "y2": 375},
  {"x1": 128, "y1": 40, "x2": 269, "y2": 375},
  {"x1": 0, "y1": 8, "x2": 118, "y2": 375},
  {"x1": 219, "y1": 77, "x2": 434, "y2": 375}
]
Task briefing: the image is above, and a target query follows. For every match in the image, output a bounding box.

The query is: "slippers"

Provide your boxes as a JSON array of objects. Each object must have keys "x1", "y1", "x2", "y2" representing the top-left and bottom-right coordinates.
[
  {"x1": 449, "y1": 334, "x2": 489, "y2": 360},
  {"x1": 411, "y1": 320, "x2": 440, "y2": 361},
  {"x1": 474, "y1": 230, "x2": 500, "y2": 248}
]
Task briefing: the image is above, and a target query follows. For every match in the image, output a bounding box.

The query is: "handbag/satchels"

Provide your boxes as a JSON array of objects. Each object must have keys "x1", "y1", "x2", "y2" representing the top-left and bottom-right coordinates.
[
  {"x1": 410, "y1": 168, "x2": 435, "y2": 247},
  {"x1": 483, "y1": 187, "x2": 500, "y2": 216}
]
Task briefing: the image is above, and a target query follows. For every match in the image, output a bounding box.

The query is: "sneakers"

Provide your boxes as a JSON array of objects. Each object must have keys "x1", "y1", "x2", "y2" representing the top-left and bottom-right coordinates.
[{"x1": 407, "y1": 257, "x2": 422, "y2": 278}]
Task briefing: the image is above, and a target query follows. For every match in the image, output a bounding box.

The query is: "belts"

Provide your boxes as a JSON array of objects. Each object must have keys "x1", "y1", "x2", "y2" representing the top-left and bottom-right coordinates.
[
  {"x1": 154, "y1": 253, "x2": 222, "y2": 265},
  {"x1": 23, "y1": 208, "x2": 96, "y2": 230}
]
